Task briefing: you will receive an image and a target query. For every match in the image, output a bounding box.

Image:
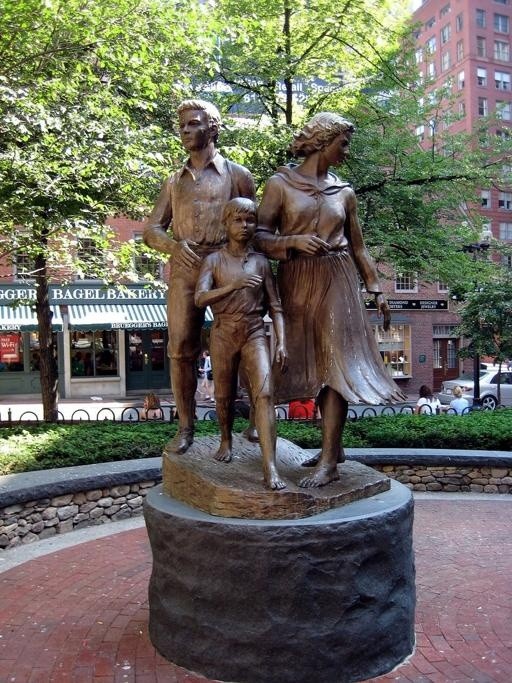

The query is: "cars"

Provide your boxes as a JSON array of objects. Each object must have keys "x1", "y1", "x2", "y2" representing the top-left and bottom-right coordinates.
[{"x1": 435, "y1": 368, "x2": 512, "y2": 412}]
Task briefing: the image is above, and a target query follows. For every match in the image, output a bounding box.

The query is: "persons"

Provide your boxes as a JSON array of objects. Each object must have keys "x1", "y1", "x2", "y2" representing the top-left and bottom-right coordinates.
[
  {"x1": 207, "y1": 393, "x2": 250, "y2": 420},
  {"x1": 286, "y1": 396, "x2": 319, "y2": 418},
  {"x1": 194, "y1": 195, "x2": 290, "y2": 490},
  {"x1": 196, "y1": 349, "x2": 214, "y2": 402},
  {"x1": 414, "y1": 384, "x2": 444, "y2": 415},
  {"x1": 137, "y1": 391, "x2": 164, "y2": 421},
  {"x1": 148, "y1": 98, "x2": 257, "y2": 456},
  {"x1": 445, "y1": 385, "x2": 471, "y2": 415},
  {"x1": 255, "y1": 112, "x2": 393, "y2": 488}
]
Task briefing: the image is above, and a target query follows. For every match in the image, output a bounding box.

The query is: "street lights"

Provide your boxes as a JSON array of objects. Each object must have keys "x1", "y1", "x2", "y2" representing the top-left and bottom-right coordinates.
[{"x1": 456, "y1": 241, "x2": 491, "y2": 411}]
